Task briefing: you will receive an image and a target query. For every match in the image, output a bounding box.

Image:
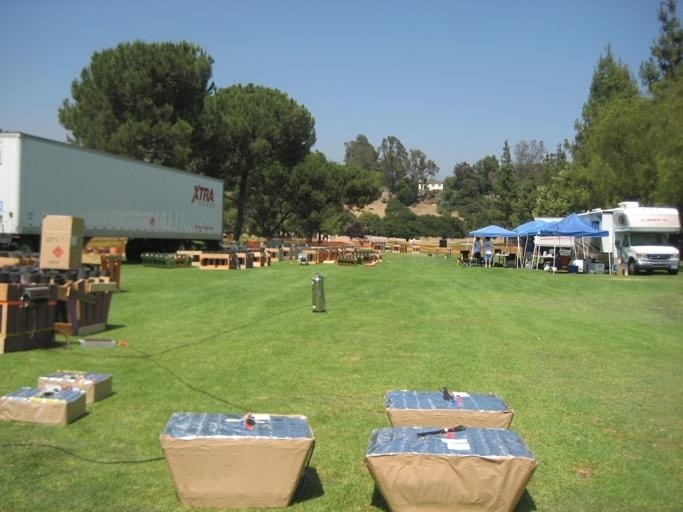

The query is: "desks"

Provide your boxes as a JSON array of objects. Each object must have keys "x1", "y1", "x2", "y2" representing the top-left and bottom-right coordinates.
[
  {"x1": 436, "y1": 247, "x2": 451, "y2": 256},
  {"x1": 531, "y1": 254, "x2": 557, "y2": 271}
]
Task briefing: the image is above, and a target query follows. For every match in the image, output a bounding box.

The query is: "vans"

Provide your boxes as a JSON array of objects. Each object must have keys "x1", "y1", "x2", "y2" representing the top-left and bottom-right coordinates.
[{"x1": 601, "y1": 201, "x2": 681, "y2": 274}]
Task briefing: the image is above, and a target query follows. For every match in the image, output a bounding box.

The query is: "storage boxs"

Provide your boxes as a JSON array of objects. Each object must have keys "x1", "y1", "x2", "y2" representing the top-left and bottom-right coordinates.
[{"x1": 39, "y1": 214, "x2": 84, "y2": 270}]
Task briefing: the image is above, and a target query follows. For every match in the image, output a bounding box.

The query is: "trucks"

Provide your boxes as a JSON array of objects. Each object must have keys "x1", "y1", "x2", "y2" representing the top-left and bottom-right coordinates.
[{"x1": 0, "y1": 131, "x2": 225, "y2": 260}]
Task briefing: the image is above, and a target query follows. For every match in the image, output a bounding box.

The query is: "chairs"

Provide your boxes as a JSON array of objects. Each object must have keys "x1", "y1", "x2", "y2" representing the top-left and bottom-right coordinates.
[{"x1": 459, "y1": 249, "x2": 517, "y2": 268}]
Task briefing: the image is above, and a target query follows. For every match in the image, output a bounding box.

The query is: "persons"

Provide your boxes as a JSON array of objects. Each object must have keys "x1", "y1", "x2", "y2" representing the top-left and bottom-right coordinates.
[
  {"x1": 474, "y1": 237, "x2": 481, "y2": 264},
  {"x1": 483, "y1": 237, "x2": 494, "y2": 267}
]
